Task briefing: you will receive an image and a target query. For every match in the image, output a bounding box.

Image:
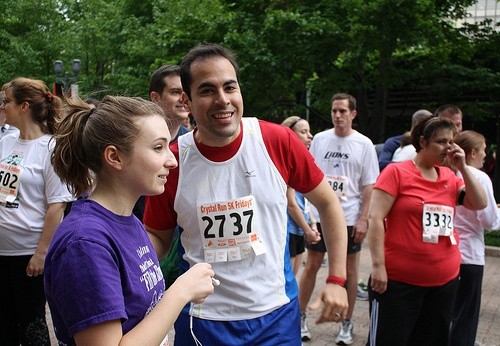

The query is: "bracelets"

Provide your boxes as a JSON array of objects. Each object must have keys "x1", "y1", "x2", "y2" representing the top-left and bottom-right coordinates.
[{"x1": 326, "y1": 276, "x2": 346, "y2": 287}]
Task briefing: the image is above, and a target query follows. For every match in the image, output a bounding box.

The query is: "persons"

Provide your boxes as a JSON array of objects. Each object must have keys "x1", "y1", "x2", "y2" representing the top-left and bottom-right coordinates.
[{"x1": 0, "y1": 44, "x2": 500, "y2": 346}]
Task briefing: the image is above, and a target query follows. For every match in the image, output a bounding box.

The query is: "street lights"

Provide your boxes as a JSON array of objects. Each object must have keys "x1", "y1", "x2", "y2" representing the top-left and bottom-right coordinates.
[{"x1": 53, "y1": 59, "x2": 81, "y2": 93}]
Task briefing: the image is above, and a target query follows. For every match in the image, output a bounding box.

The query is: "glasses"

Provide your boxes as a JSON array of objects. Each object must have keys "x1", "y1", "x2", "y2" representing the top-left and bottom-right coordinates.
[{"x1": 3, "y1": 99, "x2": 15, "y2": 104}]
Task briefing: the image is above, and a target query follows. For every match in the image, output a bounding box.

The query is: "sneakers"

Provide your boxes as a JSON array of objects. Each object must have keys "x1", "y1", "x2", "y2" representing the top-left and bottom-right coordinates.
[
  {"x1": 335, "y1": 319, "x2": 353, "y2": 345},
  {"x1": 356, "y1": 282, "x2": 368, "y2": 298},
  {"x1": 300, "y1": 313, "x2": 312, "y2": 341}
]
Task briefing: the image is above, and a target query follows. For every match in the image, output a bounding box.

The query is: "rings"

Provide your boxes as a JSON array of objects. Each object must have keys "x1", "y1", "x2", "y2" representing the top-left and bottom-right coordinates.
[{"x1": 336, "y1": 312, "x2": 341, "y2": 317}]
